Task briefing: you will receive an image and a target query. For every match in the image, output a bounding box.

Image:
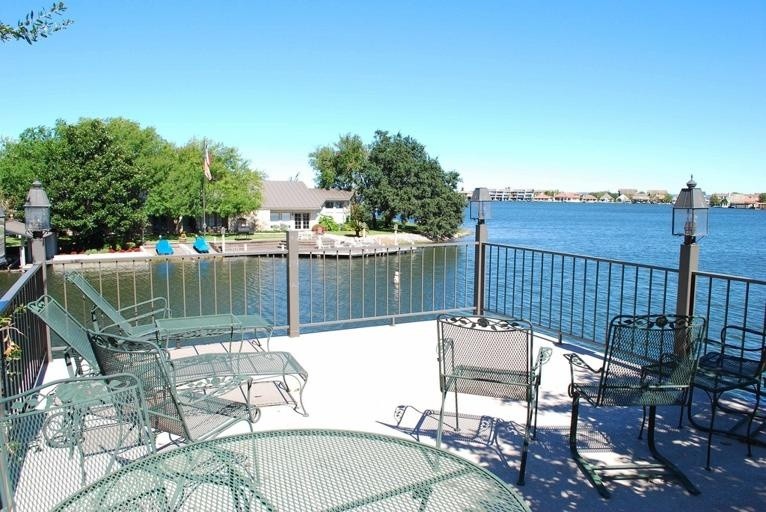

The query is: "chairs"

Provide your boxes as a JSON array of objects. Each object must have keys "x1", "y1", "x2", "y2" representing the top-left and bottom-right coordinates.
[
  {"x1": 28, "y1": 294, "x2": 309, "y2": 416},
  {"x1": 643, "y1": 306, "x2": 766, "y2": 469},
  {"x1": 564, "y1": 314, "x2": 706, "y2": 498},
  {"x1": 3, "y1": 370, "x2": 171, "y2": 511},
  {"x1": 67, "y1": 273, "x2": 275, "y2": 366},
  {"x1": 438, "y1": 315, "x2": 552, "y2": 487}
]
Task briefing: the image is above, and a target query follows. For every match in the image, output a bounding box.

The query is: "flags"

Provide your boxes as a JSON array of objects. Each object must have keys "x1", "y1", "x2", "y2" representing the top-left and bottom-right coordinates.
[{"x1": 203, "y1": 147, "x2": 213, "y2": 181}]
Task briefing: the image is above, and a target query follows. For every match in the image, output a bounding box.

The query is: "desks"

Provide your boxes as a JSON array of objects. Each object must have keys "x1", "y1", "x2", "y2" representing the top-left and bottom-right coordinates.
[{"x1": 45, "y1": 429, "x2": 530, "y2": 512}]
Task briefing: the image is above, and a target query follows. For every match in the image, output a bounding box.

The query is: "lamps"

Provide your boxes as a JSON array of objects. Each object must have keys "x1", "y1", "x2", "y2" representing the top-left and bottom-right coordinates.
[
  {"x1": 469, "y1": 187, "x2": 493, "y2": 222},
  {"x1": 25, "y1": 180, "x2": 51, "y2": 236},
  {"x1": 673, "y1": 174, "x2": 709, "y2": 243}
]
